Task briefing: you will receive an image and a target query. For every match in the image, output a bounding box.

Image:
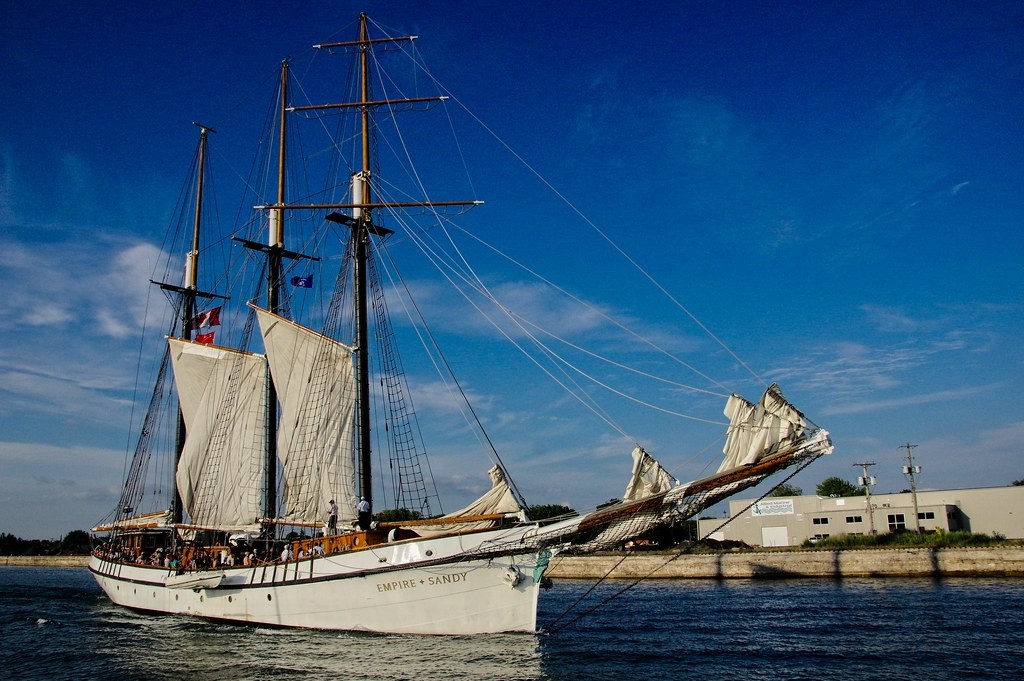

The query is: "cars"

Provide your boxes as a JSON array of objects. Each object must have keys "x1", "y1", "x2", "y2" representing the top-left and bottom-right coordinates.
[{"x1": 808, "y1": 537, "x2": 823, "y2": 544}]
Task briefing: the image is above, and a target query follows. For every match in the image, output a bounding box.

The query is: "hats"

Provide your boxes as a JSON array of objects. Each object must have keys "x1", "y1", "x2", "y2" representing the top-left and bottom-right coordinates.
[
  {"x1": 284, "y1": 545, "x2": 289, "y2": 549},
  {"x1": 245, "y1": 552, "x2": 249, "y2": 555},
  {"x1": 329, "y1": 500, "x2": 334, "y2": 504},
  {"x1": 361, "y1": 496, "x2": 365, "y2": 500}
]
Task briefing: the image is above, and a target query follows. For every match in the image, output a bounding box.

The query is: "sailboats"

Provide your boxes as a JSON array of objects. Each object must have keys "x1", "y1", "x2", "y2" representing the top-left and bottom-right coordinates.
[{"x1": 84, "y1": 10, "x2": 833, "y2": 634}]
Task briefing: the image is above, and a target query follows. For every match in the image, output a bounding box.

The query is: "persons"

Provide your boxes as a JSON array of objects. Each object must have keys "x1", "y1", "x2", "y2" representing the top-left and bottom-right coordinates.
[
  {"x1": 357, "y1": 496, "x2": 369, "y2": 530},
  {"x1": 102, "y1": 542, "x2": 181, "y2": 569},
  {"x1": 326, "y1": 500, "x2": 338, "y2": 536},
  {"x1": 187, "y1": 542, "x2": 325, "y2": 569}
]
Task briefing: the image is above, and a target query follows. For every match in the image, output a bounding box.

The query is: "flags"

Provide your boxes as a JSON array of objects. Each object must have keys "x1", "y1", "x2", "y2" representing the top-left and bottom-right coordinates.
[
  {"x1": 290, "y1": 274, "x2": 314, "y2": 288},
  {"x1": 195, "y1": 330, "x2": 214, "y2": 345},
  {"x1": 191, "y1": 305, "x2": 221, "y2": 331}
]
